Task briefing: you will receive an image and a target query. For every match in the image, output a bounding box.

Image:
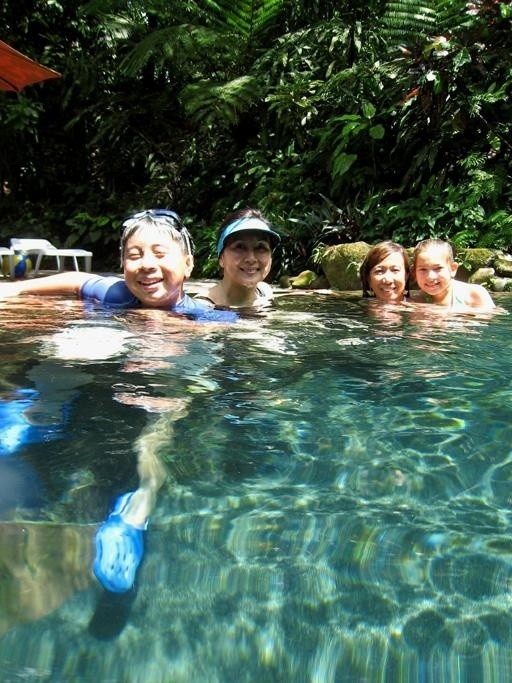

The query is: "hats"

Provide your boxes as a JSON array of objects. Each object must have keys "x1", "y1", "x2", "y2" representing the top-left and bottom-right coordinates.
[{"x1": 217, "y1": 216, "x2": 283, "y2": 256}]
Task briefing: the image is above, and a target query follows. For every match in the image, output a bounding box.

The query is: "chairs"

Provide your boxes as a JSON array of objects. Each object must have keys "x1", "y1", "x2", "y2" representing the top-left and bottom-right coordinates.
[{"x1": 9, "y1": 236, "x2": 92, "y2": 276}]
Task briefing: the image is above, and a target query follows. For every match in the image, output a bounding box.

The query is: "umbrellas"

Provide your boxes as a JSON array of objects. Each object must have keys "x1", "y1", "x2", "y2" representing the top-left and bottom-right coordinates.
[{"x1": 0, "y1": 37, "x2": 64, "y2": 93}]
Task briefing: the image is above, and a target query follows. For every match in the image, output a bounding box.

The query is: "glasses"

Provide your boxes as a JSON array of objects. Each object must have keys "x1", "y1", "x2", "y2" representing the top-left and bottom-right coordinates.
[{"x1": 121, "y1": 207, "x2": 186, "y2": 233}]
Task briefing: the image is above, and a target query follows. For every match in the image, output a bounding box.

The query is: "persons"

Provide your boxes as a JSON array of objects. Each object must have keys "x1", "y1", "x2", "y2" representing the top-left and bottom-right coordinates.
[
  {"x1": 191, "y1": 206, "x2": 314, "y2": 327},
  {"x1": 358, "y1": 236, "x2": 418, "y2": 336},
  {"x1": 0, "y1": 205, "x2": 285, "y2": 592},
  {"x1": 413, "y1": 233, "x2": 509, "y2": 331}
]
